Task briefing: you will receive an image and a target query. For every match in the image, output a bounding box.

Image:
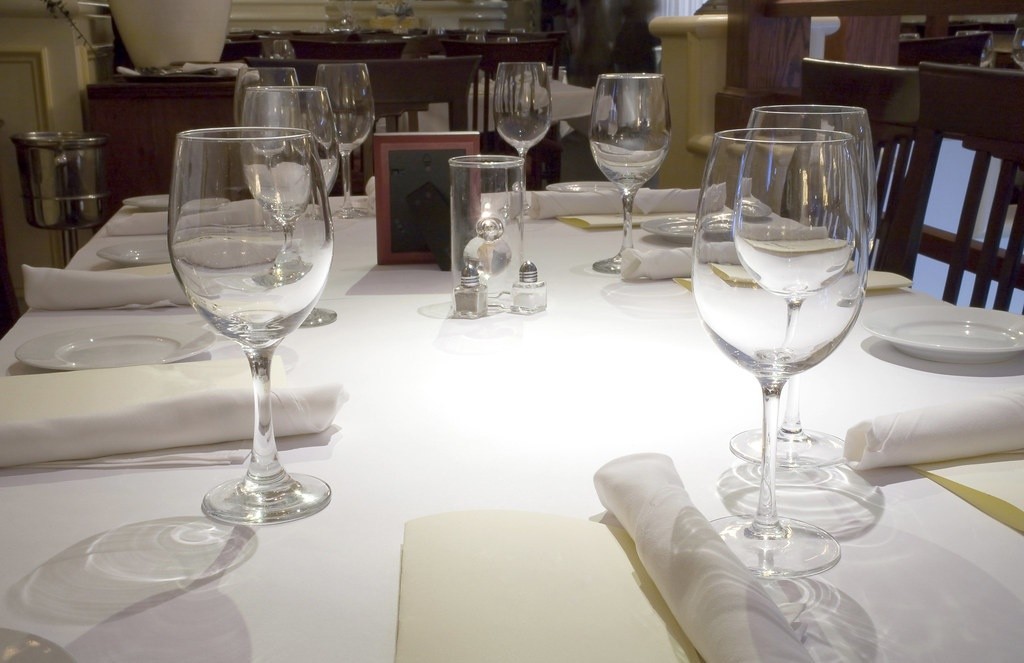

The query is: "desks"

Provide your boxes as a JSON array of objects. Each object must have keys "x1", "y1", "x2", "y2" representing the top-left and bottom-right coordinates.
[
  {"x1": 353, "y1": 79, "x2": 614, "y2": 189},
  {"x1": 0, "y1": 191, "x2": 1024, "y2": 663}
]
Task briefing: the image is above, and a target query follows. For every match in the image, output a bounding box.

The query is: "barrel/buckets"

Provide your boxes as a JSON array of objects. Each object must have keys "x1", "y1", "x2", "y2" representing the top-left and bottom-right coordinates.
[{"x1": 9, "y1": 131, "x2": 109, "y2": 230}]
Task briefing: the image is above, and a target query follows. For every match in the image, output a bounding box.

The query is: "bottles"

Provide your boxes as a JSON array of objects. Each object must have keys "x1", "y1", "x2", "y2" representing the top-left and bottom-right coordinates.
[
  {"x1": 453, "y1": 262, "x2": 489, "y2": 319},
  {"x1": 511, "y1": 260, "x2": 547, "y2": 316}
]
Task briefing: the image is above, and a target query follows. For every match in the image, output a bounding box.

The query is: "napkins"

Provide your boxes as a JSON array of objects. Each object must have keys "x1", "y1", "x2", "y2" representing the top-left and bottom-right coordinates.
[
  {"x1": 741, "y1": 223, "x2": 829, "y2": 243},
  {"x1": 592, "y1": 453, "x2": 814, "y2": 663},
  {"x1": 0, "y1": 353, "x2": 347, "y2": 469},
  {"x1": 528, "y1": 181, "x2": 728, "y2": 220},
  {"x1": 105, "y1": 199, "x2": 261, "y2": 238},
  {"x1": 21, "y1": 262, "x2": 195, "y2": 311},
  {"x1": 842, "y1": 387, "x2": 1024, "y2": 471},
  {"x1": 618, "y1": 241, "x2": 741, "y2": 283},
  {"x1": 172, "y1": 235, "x2": 303, "y2": 269}
]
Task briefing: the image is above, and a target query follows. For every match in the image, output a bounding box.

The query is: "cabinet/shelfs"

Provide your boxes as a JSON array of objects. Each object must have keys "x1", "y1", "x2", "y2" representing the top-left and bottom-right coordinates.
[{"x1": 84, "y1": 59, "x2": 253, "y2": 229}]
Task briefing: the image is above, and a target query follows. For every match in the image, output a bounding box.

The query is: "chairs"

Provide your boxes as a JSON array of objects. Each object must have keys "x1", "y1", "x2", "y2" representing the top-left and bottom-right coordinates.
[
  {"x1": 221, "y1": 30, "x2": 571, "y2": 194},
  {"x1": 780, "y1": 56, "x2": 920, "y2": 271},
  {"x1": 881, "y1": 60, "x2": 1024, "y2": 316},
  {"x1": 898, "y1": 32, "x2": 992, "y2": 67}
]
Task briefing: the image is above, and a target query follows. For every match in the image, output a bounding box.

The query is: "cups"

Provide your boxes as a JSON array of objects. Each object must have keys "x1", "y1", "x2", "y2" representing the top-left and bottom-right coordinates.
[
  {"x1": 448, "y1": 154, "x2": 527, "y2": 313},
  {"x1": 467, "y1": 34, "x2": 486, "y2": 43},
  {"x1": 273, "y1": 39, "x2": 292, "y2": 60},
  {"x1": 498, "y1": 36, "x2": 517, "y2": 43},
  {"x1": 428, "y1": 26, "x2": 446, "y2": 35}
]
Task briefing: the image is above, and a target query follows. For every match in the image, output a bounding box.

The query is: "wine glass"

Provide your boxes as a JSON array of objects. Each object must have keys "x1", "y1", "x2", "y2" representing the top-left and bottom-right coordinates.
[
  {"x1": 231, "y1": 66, "x2": 301, "y2": 233},
  {"x1": 316, "y1": 61, "x2": 375, "y2": 220},
  {"x1": 691, "y1": 126, "x2": 870, "y2": 580},
  {"x1": 241, "y1": 86, "x2": 338, "y2": 329},
  {"x1": 493, "y1": 62, "x2": 554, "y2": 222},
  {"x1": 168, "y1": 126, "x2": 332, "y2": 528},
  {"x1": 732, "y1": 103, "x2": 879, "y2": 470},
  {"x1": 587, "y1": 73, "x2": 672, "y2": 275}
]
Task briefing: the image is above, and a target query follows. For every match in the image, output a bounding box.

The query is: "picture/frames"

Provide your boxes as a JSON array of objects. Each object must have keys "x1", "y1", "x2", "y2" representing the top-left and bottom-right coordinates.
[{"x1": 372, "y1": 131, "x2": 481, "y2": 266}]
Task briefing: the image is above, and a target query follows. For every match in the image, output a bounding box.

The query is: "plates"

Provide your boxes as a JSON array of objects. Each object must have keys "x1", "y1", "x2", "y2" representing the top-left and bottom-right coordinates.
[
  {"x1": 860, "y1": 305, "x2": 1024, "y2": 363},
  {"x1": 122, "y1": 193, "x2": 169, "y2": 211},
  {"x1": 14, "y1": 322, "x2": 217, "y2": 371},
  {"x1": 97, "y1": 239, "x2": 171, "y2": 266},
  {"x1": 641, "y1": 215, "x2": 734, "y2": 244}
]
